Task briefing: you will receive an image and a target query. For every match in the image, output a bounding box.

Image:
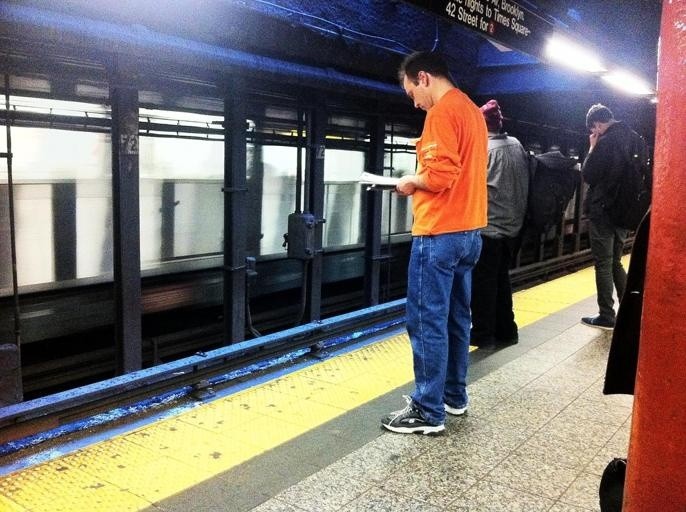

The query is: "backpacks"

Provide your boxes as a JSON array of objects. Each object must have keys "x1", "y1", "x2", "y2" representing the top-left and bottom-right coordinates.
[{"x1": 610, "y1": 131, "x2": 652, "y2": 230}]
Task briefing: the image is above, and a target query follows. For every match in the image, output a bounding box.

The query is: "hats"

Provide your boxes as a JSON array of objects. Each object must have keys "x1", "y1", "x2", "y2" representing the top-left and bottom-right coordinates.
[{"x1": 480, "y1": 99, "x2": 503, "y2": 131}]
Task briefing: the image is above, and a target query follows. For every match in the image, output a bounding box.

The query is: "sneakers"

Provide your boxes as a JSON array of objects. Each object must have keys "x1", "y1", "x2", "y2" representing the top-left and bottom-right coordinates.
[
  {"x1": 470, "y1": 324, "x2": 517, "y2": 347},
  {"x1": 581, "y1": 317, "x2": 615, "y2": 330},
  {"x1": 381, "y1": 403, "x2": 446, "y2": 436},
  {"x1": 402, "y1": 394, "x2": 467, "y2": 416}
]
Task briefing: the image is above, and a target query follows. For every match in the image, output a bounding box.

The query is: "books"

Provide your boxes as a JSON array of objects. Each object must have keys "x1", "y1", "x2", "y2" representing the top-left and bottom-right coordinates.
[{"x1": 358, "y1": 171, "x2": 398, "y2": 190}]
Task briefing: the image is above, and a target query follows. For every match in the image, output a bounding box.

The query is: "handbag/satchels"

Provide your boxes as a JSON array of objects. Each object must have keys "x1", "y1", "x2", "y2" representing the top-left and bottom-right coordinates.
[{"x1": 515, "y1": 150, "x2": 580, "y2": 239}]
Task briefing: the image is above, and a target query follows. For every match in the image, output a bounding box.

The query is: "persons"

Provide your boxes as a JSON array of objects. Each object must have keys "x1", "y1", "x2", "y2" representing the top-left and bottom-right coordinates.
[
  {"x1": 471, "y1": 98, "x2": 532, "y2": 352},
  {"x1": 579, "y1": 104, "x2": 648, "y2": 331},
  {"x1": 379, "y1": 51, "x2": 490, "y2": 436}
]
